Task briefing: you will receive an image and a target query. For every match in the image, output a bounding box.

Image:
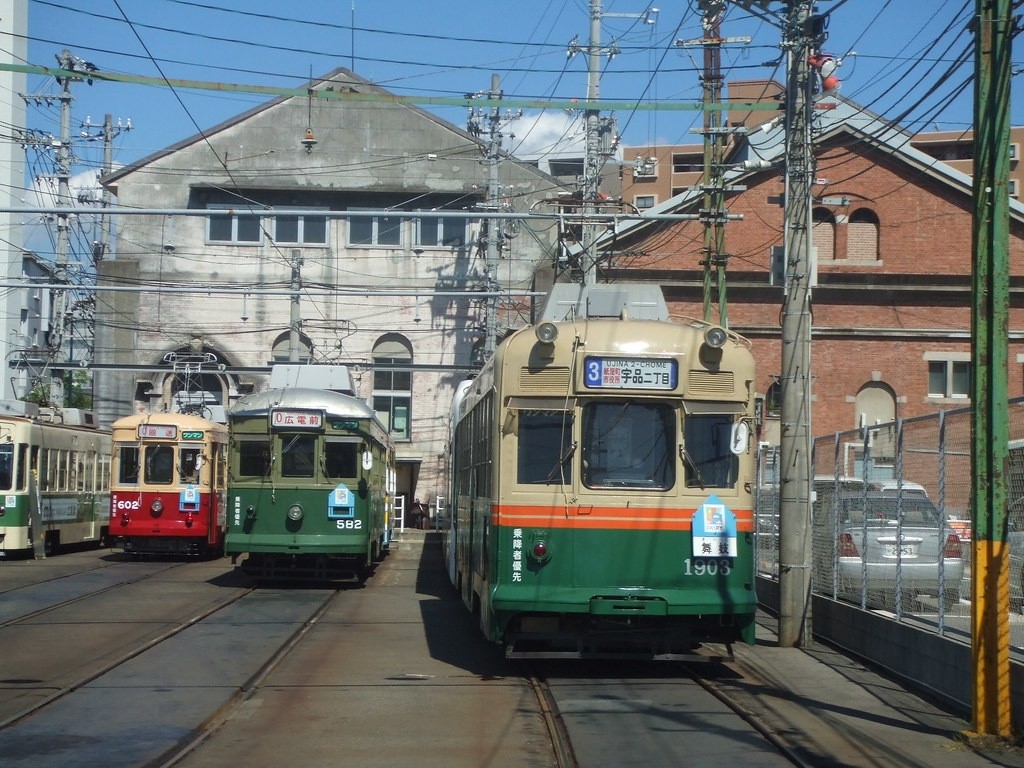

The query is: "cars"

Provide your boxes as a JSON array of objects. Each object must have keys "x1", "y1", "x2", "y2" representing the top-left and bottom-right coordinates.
[
  {"x1": 755, "y1": 489, "x2": 783, "y2": 549},
  {"x1": 872, "y1": 479, "x2": 937, "y2": 527},
  {"x1": 812, "y1": 491, "x2": 963, "y2": 612}
]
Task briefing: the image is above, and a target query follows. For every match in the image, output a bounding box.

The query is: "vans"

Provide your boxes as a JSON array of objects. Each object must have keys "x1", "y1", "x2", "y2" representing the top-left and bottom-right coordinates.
[
  {"x1": 961, "y1": 439, "x2": 1024, "y2": 607},
  {"x1": 813, "y1": 474, "x2": 875, "y2": 509}
]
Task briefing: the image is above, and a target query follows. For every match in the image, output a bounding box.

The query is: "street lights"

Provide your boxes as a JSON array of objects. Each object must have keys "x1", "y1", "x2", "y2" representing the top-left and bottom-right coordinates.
[
  {"x1": 581, "y1": 192, "x2": 613, "y2": 285},
  {"x1": 778, "y1": 55, "x2": 840, "y2": 649}
]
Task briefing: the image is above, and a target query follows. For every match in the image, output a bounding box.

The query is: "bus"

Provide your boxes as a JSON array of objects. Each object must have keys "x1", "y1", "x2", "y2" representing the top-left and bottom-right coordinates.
[
  {"x1": 0, "y1": 414, "x2": 113, "y2": 557},
  {"x1": 108, "y1": 413, "x2": 229, "y2": 555},
  {"x1": 224, "y1": 365, "x2": 396, "y2": 581},
  {"x1": 443, "y1": 284, "x2": 758, "y2": 659}
]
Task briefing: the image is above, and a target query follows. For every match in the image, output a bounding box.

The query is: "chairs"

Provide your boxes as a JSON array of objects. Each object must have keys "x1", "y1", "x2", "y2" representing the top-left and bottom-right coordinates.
[
  {"x1": 435, "y1": 497, "x2": 446, "y2": 529},
  {"x1": 849, "y1": 511, "x2": 864, "y2": 522},
  {"x1": 904, "y1": 511, "x2": 923, "y2": 522}
]
